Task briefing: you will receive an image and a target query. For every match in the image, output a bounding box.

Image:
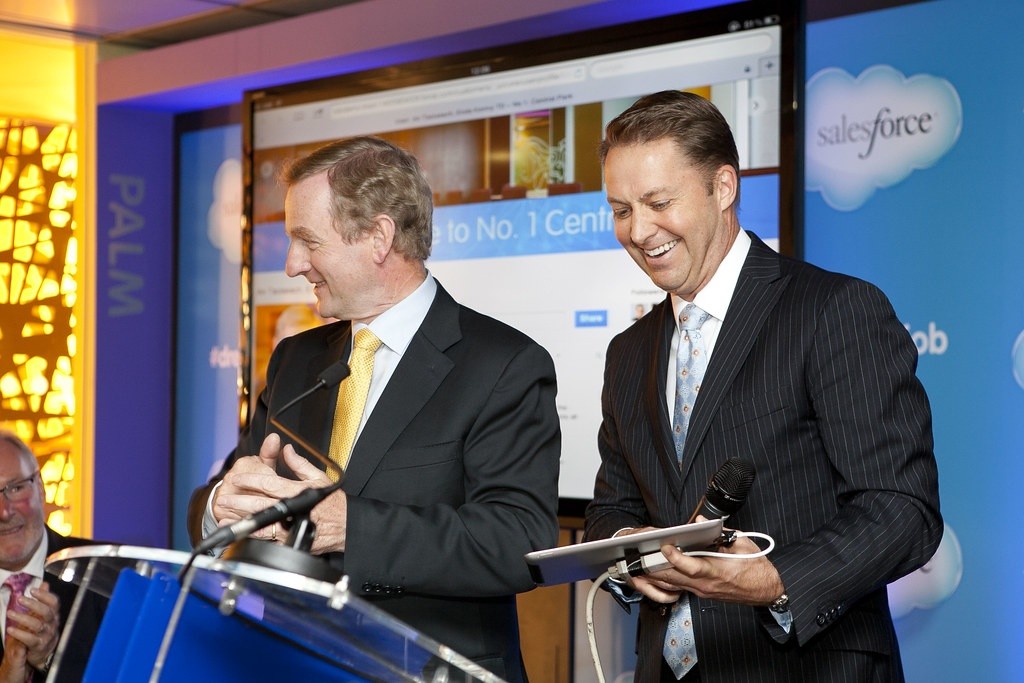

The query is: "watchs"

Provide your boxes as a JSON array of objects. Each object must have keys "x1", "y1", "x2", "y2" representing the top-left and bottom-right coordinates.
[{"x1": 769, "y1": 595, "x2": 790, "y2": 614}]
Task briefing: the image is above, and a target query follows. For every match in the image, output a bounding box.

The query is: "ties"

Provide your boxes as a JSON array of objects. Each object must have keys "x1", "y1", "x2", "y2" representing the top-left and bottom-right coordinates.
[
  {"x1": 1, "y1": 571, "x2": 36, "y2": 645},
  {"x1": 325, "y1": 327, "x2": 384, "y2": 498},
  {"x1": 662, "y1": 303, "x2": 710, "y2": 681}
]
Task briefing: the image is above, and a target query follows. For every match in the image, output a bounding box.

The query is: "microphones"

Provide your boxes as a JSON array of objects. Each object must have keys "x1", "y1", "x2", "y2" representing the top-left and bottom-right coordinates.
[
  {"x1": 199, "y1": 359, "x2": 351, "y2": 550},
  {"x1": 641, "y1": 457, "x2": 756, "y2": 612}
]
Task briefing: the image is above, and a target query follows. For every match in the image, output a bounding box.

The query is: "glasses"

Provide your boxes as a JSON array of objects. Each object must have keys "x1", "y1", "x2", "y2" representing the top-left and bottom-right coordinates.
[{"x1": 0, "y1": 468, "x2": 43, "y2": 504}]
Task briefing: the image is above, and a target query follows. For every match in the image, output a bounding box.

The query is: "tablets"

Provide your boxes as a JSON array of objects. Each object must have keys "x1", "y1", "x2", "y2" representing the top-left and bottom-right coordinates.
[{"x1": 523, "y1": 519, "x2": 723, "y2": 587}]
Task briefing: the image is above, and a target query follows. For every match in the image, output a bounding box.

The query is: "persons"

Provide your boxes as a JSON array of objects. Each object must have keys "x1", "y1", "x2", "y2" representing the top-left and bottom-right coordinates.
[
  {"x1": 187, "y1": 137, "x2": 563, "y2": 683},
  {"x1": 0, "y1": 434, "x2": 133, "y2": 683},
  {"x1": 583, "y1": 89, "x2": 945, "y2": 683}
]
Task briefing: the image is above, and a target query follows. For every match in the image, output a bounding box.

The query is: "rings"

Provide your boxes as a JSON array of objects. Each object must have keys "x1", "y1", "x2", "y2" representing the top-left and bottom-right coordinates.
[{"x1": 272, "y1": 524, "x2": 276, "y2": 540}]
